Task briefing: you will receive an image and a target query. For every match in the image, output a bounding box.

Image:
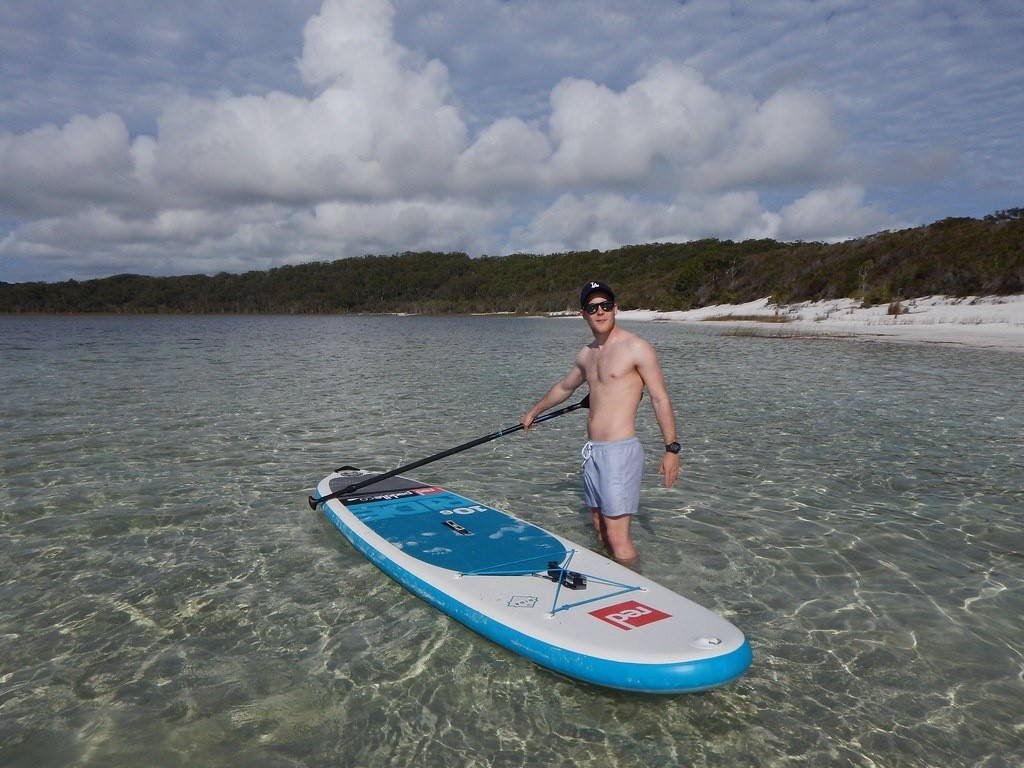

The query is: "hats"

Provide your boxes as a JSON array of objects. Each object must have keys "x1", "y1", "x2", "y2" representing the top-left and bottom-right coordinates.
[{"x1": 579, "y1": 280, "x2": 614, "y2": 308}]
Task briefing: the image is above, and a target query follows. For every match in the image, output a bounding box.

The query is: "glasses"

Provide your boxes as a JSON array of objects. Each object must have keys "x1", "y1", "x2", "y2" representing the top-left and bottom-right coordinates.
[{"x1": 583, "y1": 299, "x2": 615, "y2": 314}]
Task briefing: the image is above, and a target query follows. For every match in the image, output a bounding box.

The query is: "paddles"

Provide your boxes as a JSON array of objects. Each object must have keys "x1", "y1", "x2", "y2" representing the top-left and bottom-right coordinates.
[{"x1": 306, "y1": 391, "x2": 646, "y2": 510}]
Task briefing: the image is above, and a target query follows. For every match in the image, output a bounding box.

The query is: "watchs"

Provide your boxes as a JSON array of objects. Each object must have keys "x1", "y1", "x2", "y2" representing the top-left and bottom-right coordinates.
[{"x1": 666, "y1": 441, "x2": 681, "y2": 454}]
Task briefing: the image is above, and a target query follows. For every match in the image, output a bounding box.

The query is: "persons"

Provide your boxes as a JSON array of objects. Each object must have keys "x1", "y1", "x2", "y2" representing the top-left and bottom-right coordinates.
[{"x1": 520, "y1": 281, "x2": 682, "y2": 558}]
